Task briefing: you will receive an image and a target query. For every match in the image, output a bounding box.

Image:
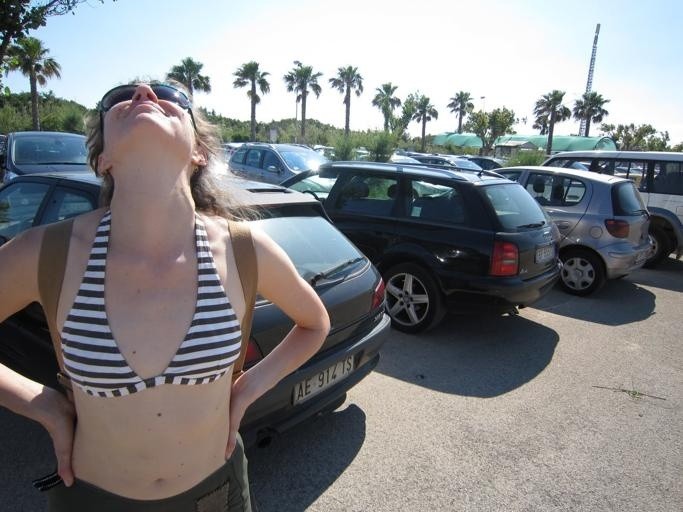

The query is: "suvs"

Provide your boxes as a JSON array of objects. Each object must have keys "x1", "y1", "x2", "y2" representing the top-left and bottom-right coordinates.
[
  {"x1": 0, "y1": 170, "x2": 392, "y2": 450},
  {"x1": 227, "y1": 142, "x2": 338, "y2": 195},
  {"x1": 409, "y1": 155, "x2": 484, "y2": 173},
  {"x1": 278, "y1": 159, "x2": 564, "y2": 334},
  {"x1": 489, "y1": 164, "x2": 652, "y2": 297}
]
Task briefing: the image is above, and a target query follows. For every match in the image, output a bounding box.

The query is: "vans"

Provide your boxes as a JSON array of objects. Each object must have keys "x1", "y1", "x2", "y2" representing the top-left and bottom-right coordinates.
[{"x1": 540, "y1": 150, "x2": 683, "y2": 269}]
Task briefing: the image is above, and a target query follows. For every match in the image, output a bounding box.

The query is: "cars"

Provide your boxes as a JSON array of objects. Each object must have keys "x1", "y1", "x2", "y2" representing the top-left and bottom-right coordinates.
[
  {"x1": 2, "y1": 130, "x2": 95, "y2": 185},
  {"x1": 360, "y1": 155, "x2": 422, "y2": 164}
]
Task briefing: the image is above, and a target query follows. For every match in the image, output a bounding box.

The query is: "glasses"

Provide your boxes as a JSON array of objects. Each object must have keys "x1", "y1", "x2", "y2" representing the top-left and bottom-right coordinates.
[{"x1": 103, "y1": 87, "x2": 198, "y2": 137}]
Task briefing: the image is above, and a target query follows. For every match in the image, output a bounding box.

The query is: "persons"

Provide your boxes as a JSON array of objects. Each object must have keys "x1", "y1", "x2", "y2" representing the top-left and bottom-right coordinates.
[{"x1": 0, "y1": 80, "x2": 331, "y2": 512}]
